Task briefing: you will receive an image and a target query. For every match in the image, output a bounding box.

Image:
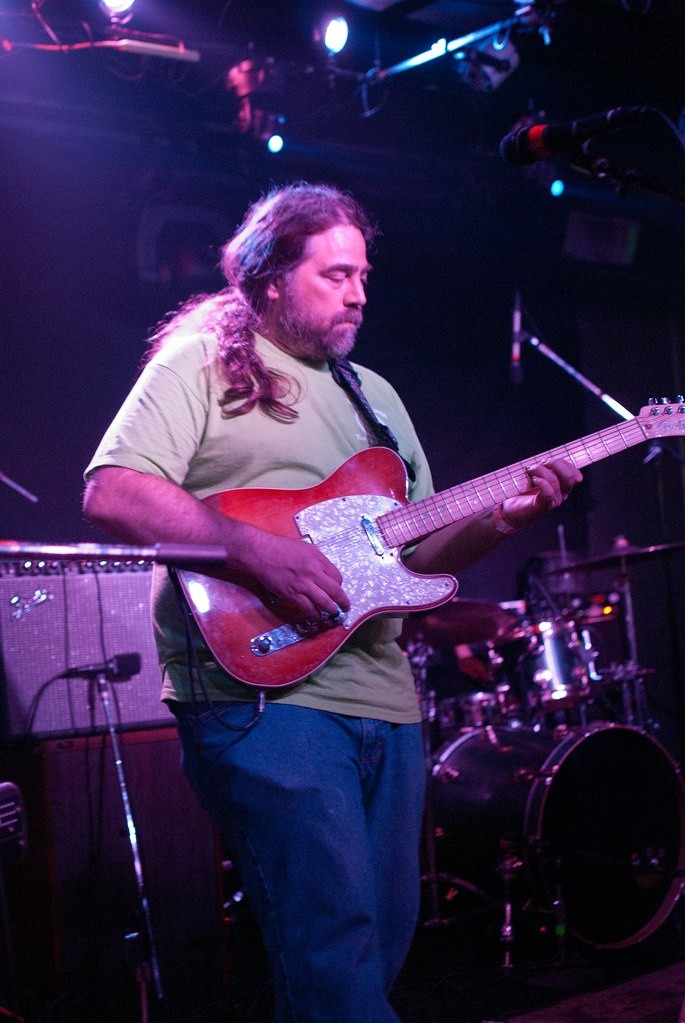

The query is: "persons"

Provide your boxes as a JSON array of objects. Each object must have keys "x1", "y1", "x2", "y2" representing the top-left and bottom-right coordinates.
[{"x1": 82, "y1": 184, "x2": 584, "y2": 1023}]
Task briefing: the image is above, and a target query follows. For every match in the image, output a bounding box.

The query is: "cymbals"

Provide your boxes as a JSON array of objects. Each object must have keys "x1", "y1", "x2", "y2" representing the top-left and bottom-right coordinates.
[
  {"x1": 546, "y1": 532, "x2": 685, "y2": 578},
  {"x1": 395, "y1": 596, "x2": 521, "y2": 651}
]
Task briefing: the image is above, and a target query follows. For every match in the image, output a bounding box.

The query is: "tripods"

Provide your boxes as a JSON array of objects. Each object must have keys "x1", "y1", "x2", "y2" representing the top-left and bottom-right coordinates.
[{"x1": 404, "y1": 636, "x2": 549, "y2": 938}]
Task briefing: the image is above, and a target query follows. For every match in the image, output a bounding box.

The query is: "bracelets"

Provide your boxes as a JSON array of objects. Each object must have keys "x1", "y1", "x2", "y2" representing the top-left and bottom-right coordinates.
[{"x1": 492, "y1": 502, "x2": 526, "y2": 535}]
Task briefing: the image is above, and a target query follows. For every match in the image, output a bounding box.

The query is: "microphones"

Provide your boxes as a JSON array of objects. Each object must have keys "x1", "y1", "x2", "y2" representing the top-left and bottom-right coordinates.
[
  {"x1": 507, "y1": 297, "x2": 523, "y2": 384},
  {"x1": 500, "y1": 106, "x2": 656, "y2": 167},
  {"x1": 64, "y1": 654, "x2": 140, "y2": 677},
  {"x1": 523, "y1": 576, "x2": 538, "y2": 616}
]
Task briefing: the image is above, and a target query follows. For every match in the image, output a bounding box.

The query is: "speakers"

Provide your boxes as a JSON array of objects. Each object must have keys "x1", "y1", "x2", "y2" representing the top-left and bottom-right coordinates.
[
  {"x1": 0, "y1": 541, "x2": 179, "y2": 736},
  {"x1": 16, "y1": 726, "x2": 240, "y2": 1023}
]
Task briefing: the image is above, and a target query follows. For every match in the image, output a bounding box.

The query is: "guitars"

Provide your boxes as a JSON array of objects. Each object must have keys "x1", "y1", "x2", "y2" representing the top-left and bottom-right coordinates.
[{"x1": 159, "y1": 390, "x2": 685, "y2": 701}]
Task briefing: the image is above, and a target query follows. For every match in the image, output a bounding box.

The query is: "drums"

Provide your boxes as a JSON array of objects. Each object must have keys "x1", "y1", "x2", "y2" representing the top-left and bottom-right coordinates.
[
  {"x1": 569, "y1": 605, "x2": 629, "y2": 686},
  {"x1": 426, "y1": 718, "x2": 683, "y2": 954},
  {"x1": 502, "y1": 618, "x2": 595, "y2": 716}
]
